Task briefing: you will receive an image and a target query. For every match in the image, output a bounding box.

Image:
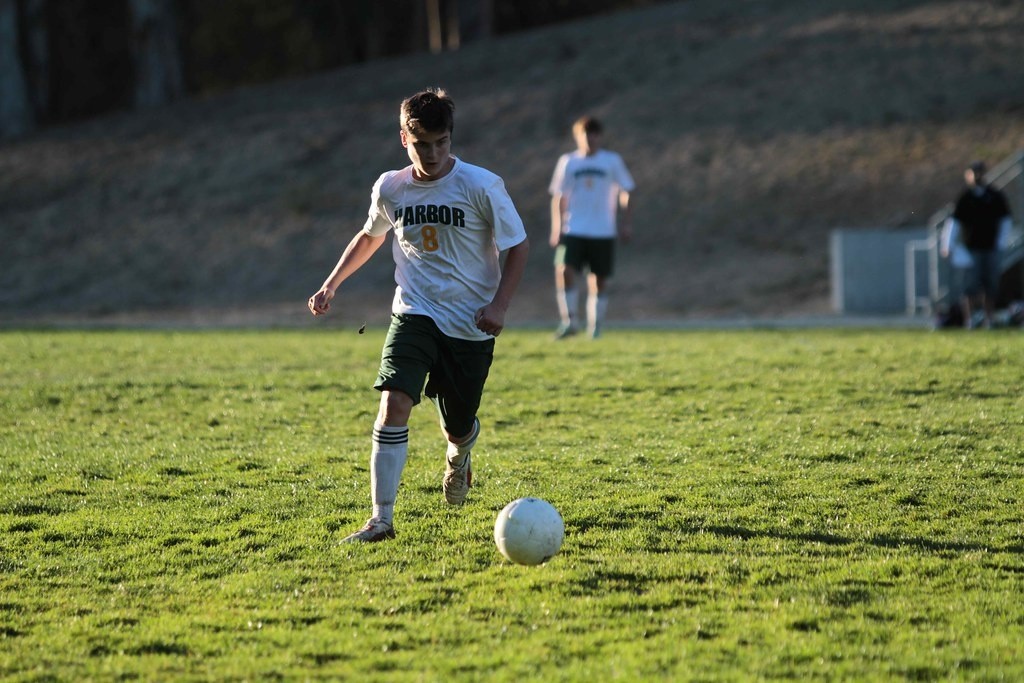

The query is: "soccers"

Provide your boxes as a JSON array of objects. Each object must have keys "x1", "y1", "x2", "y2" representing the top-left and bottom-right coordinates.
[{"x1": 493, "y1": 497, "x2": 564, "y2": 566}]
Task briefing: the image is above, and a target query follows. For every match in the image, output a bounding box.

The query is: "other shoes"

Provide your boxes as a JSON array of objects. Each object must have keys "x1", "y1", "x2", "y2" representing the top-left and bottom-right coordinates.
[
  {"x1": 589, "y1": 327, "x2": 602, "y2": 339},
  {"x1": 556, "y1": 319, "x2": 581, "y2": 339}
]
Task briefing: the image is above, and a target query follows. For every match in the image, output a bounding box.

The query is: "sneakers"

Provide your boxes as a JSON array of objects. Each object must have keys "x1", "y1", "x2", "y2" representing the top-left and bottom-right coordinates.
[
  {"x1": 338, "y1": 519, "x2": 395, "y2": 544},
  {"x1": 443, "y1": 452, "x2": 471, "y2": 504}
]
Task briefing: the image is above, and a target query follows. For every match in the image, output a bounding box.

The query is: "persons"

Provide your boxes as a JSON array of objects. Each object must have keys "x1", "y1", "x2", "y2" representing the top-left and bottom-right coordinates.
[
  {"x1": 311, "y1": 89, "x2": 527, "y2": 544},
  {"x1": 944, "y1": 157, "x2": 1015, "y2": 333},
  {"x1": 548, "y1": 113, "x2": 638, "y2": 341}
]
